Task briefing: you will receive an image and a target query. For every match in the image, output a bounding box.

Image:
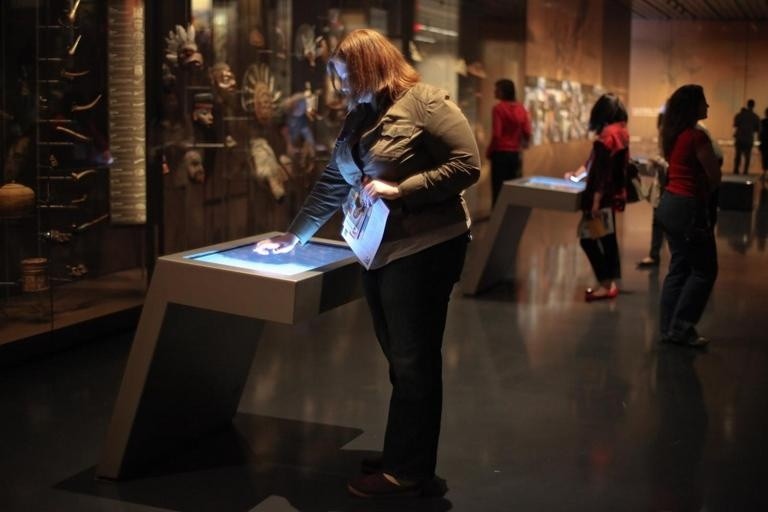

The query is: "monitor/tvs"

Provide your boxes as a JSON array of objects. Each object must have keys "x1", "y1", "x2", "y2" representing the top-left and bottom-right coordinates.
[
  {"x1": 192, "y1": 241, "x2": 356, "y2": 275},
  {"x1": 525, "y1": 177, "x2": 587, "y2": 190}
]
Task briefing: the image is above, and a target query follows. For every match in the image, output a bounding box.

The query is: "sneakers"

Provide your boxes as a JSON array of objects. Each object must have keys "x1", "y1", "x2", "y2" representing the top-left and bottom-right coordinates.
[
  {"x1": 344, "y1": 472, "x2": 422, "y2": 499},
  {"x1": 584, "y1": 255, "x2": 712, "y2": 348}
]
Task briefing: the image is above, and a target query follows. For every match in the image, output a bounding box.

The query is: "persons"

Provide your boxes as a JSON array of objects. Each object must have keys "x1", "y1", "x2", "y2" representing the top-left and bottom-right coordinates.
[
  {"x1": 630, "y1": 114, "x2": 670, "y2": 269},
  {"x1": 253, "y1": 29, "x2": 482, "y2": 501},
  {"x1": 658, "y1": 82, "x2": 724, "y2": 350},
  {"x1": 731, "y1": 98, "x2": 759, "y2": 176},
  {"x1": 758, "y1": 110, "x2": 767, "y2": 180},
  {"x1": 170, "y1": 29, "x2": 237, "y2": 186},
  {"x1": 562, "y1": 90, "x2": 629, "y2": 303},
  {"x1": 352, "y1": 194, "x2": 365, "y2": 219},
  {"x1": 484, "y1": 79, "x2": 533, "y2": 214}
]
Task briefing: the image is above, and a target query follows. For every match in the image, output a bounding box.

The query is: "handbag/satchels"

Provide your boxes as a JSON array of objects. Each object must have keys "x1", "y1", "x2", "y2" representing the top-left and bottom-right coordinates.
[{"x1": 626, "y1": 156, "x2": 642, "y2": 204}]
